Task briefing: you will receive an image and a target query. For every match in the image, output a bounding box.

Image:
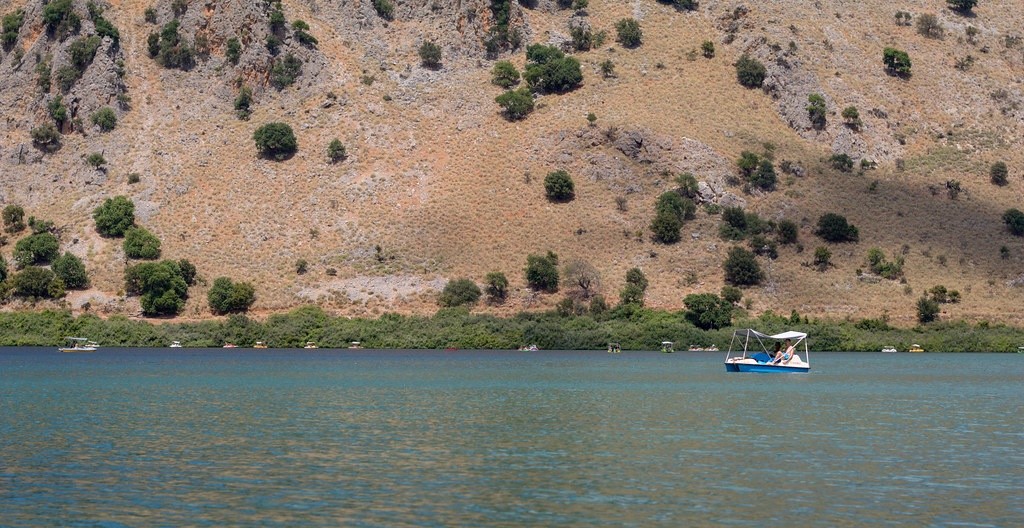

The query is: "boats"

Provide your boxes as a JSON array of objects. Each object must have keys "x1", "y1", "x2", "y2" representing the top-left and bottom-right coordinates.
[
  {"x1": 704, "y1": 348, "x2": 719, "y2": 352},
  {"x1": 448, "y1": 347, "x2": 458, "y2": 351},
  {"x1": 304, "y1": 342, "x2": 318, "y2": 349},
  {"x1": 254, "y1": 342, "x2": 267, "y2": 348},
  {"x1": 85, "y1": 340, "x2": 100, "y2": 348},
  {"x1": 170, "y1": 341, "x2": 181, "y2": 348},
  {"x1": 661, "y1": 342, "x2": 674, "y2": 353},
  {"x1": 608, "y1": 343, "x2": 621, "y2": 353},
  {"x1": 348, "y1": 342, "x2": 364, "y2": 349},
  {"x1": 63, "y1": 337, "x2": 97, "y2": 352},
  {"x1": 909, "y1": 344, "x2": 924, "y2": 353},
  {"x1": 882, "y1": 346, "x2": 897, "y2": 352},
  {"x1": 223, "y1": 341, "x2": 239, "y2": 348},
  {"x1": 724, "y1": 329, "x2": 811, "y2": 372},
  {"x1": 519, "y1": 349, "x2": 531, "y2": 352},
  {"x1": 688, "y1": 345, "x2": 703, "y2": 351},
  {"x1": 530, "y1": 346, "x2": 538, "y2": 352}
]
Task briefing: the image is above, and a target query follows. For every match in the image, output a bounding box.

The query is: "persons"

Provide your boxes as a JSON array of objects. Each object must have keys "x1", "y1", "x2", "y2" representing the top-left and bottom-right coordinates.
[
  {"x1": 767, "y1": 339, "x2": 794, "y2": 365},
  {"x1": 70, "y1": 341, "x2": 79, "y2": 348}
]
